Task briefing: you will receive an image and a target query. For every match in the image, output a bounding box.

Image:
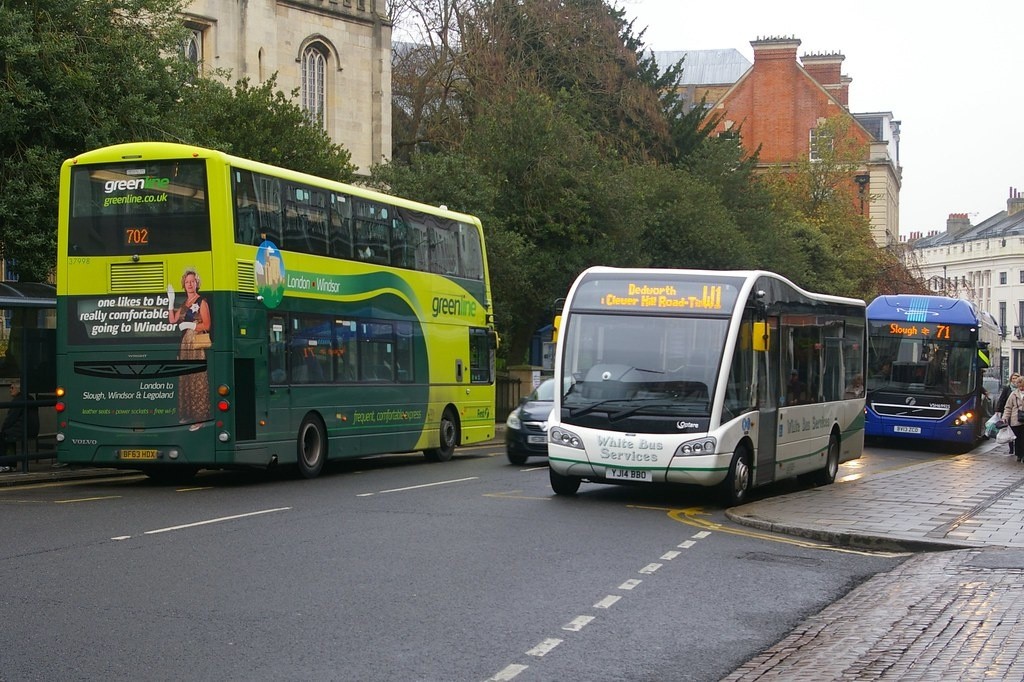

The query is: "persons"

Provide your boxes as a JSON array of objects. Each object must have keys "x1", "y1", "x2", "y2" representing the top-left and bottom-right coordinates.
[
  {"x1": 995, "y1": 373, "x2": 1024, "y2": 463},
  {"x1": 963, "y1": 369, "x2": 1000, "y2": 441},
  {"x1": 844, "y1": 372, "x2": 865, "y2": 400},
  {"x1": 166, "y1": 271, "x2": 211, "y2": 432},
  {"x1": 0, "y1": 382, "x2": 40, "y2": 472},
  {"x1": 786, "y1": 368, "x2": 833, "y2": 405},
  {"x1": 879, "y1": 359, "x2": 894, "y2": 378}
]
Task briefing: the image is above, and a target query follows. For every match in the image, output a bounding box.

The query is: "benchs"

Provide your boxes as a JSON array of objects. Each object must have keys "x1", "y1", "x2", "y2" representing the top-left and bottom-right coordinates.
[{"x1": 4, "y1": 432, "x2": 57, "y2": 472}]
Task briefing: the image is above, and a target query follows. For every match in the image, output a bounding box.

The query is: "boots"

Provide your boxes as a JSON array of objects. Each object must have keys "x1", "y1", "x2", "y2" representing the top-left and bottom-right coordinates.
[{"x1": 1009, "y1": 445, "x2": 1013, "y2": 454}]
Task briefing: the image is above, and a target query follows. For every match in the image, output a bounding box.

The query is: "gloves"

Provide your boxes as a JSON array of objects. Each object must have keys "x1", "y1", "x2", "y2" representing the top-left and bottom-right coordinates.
[
  {"x1": 168, "y1": 283, "x2": 175, "y2": 310},
  {"x1": 179, "y1": 321, "x2": 196, "y2": 331}
]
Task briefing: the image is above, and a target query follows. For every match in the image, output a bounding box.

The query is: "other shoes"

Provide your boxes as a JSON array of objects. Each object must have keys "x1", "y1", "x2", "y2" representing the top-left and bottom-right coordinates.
[
  {"x1": 1017, "y1": 457, "x2": 1024, "y2": 463},
  {"x1": 179, "y1": 418, "x2": 192, "y2": 423},
  {"x1": 189, "y1": 422, "x2": 204, "y2": 431},
  {"x1": 0, "y1": 465, "x2": 17, "y2": 473}
]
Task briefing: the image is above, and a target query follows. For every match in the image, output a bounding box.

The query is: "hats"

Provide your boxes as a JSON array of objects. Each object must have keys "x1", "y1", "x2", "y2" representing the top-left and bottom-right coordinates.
[{"x1": 790, "y1": 369, "x2": 798, "y2": 375}]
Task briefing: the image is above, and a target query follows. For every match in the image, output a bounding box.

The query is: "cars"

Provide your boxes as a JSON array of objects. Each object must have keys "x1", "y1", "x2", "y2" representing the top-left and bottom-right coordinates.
[{"x1": 503, "y1": 374, "x2": 586, "y2": 464}]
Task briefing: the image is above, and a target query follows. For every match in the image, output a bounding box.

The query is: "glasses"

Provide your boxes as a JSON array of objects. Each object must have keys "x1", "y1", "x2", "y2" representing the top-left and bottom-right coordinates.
[{"x1": 10, "y1": 386, "x2": 15, "y2": 390}]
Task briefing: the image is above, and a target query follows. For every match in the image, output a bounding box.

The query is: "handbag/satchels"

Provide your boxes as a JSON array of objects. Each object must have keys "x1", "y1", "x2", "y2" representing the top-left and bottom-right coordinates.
[
  {"x1": 984, "y1": 412, "x2": 1017, "y2": 444},
  {"x1": 1018, "y1": 410, "x2": 1024, "y2": 422},
  {"x1": 191, "y1": 324, "x2": 212, "y2": 349}
]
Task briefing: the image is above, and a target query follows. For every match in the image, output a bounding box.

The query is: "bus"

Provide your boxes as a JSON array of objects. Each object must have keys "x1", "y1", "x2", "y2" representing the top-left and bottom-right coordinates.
[
  {"x1": 544, "y1": 265, "x2": 869, "y2": 504},
  {"x1": 865, "y1": 293, "x2": 1003, "y2": 453},
  {"x1": 54, "y1": 141, "x2": 500, "y2": 484}
]
